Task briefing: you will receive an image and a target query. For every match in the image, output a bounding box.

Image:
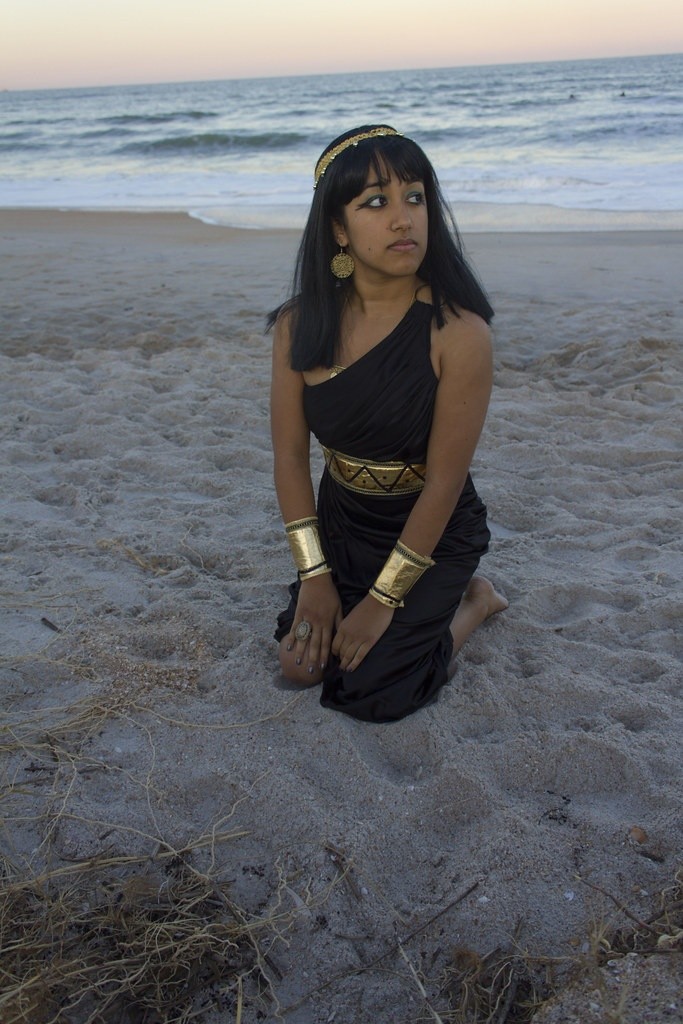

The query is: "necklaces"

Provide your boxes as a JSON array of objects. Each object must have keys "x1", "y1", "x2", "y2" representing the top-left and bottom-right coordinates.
[{"x1": 327, "y1": 281, "x2": 420, "y2": 378}]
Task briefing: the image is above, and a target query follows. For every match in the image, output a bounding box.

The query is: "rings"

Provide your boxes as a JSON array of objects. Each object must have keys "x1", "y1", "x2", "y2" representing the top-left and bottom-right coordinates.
[{"x1": 295, "y1": 621, "x2": 311, "y2": 642}]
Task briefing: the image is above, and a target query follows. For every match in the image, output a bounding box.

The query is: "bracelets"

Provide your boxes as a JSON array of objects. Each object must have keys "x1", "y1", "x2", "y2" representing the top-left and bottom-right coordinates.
[
  {"x1": 368, "y1": 539, "x2": 435, "y2": 610},
  {"x1": 282, "y1": 517, "x2": 333, "y2": 581}
]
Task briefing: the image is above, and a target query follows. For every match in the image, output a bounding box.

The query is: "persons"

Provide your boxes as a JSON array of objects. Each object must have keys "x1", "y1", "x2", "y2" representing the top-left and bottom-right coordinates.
[{"x1": 275, "y1": 124, "x2": 512, "y2": 727}]
